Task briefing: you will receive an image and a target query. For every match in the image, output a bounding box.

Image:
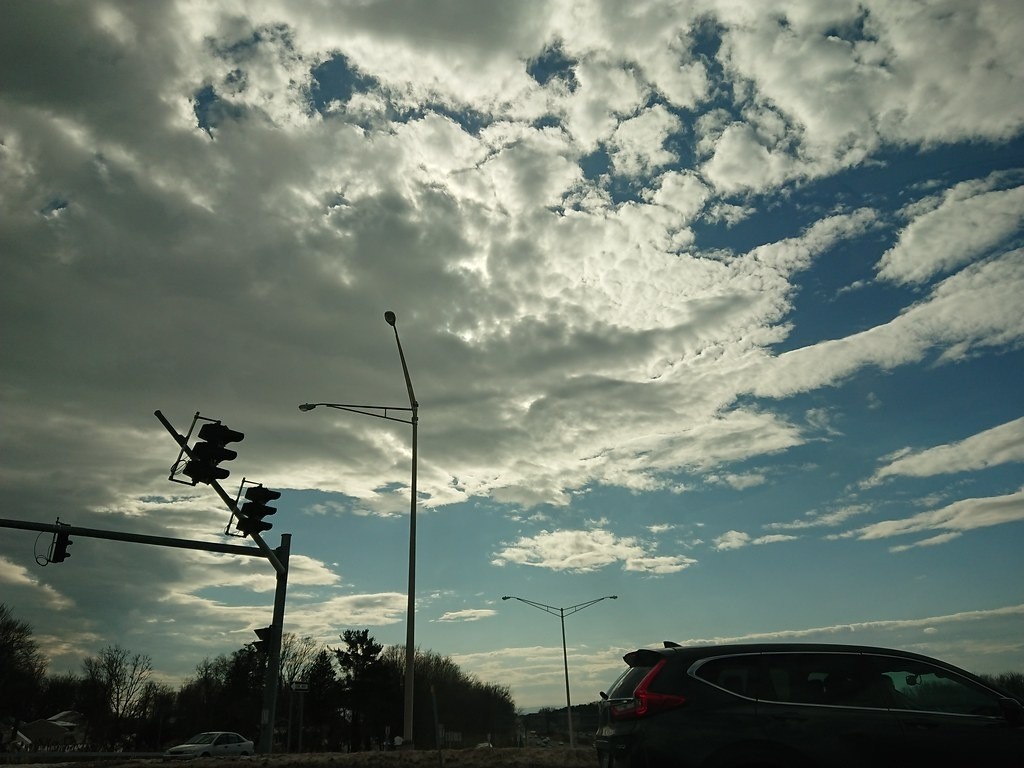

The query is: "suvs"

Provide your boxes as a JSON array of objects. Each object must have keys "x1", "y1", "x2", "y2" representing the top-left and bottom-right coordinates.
[{"x1": 593, "y1": 639, "x2": 1024, "y2": 768}]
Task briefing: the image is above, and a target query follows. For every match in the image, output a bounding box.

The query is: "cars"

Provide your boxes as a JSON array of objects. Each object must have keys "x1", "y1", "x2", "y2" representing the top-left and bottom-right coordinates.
[{"x1": 162, "y1": 730, "x2": 255, "y2": 761}]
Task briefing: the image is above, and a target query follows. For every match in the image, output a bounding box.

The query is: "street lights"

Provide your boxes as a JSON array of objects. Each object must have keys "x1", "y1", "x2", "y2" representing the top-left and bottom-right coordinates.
[
  {"x1": 502, "y1": 595, "x2": 618, "y2": 749},
  {"x1": 298, "y1": 308, "x2": 420, "y2": 750}
]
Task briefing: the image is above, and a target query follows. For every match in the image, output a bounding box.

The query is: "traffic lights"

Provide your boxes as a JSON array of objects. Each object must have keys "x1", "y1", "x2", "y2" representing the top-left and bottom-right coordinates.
[
  {"x1": 182, "y1": 423, "x2": 244, "y2": 485},
  {"x1": 235, "y1": 487, "x2": 282, "y2": 535}
]
